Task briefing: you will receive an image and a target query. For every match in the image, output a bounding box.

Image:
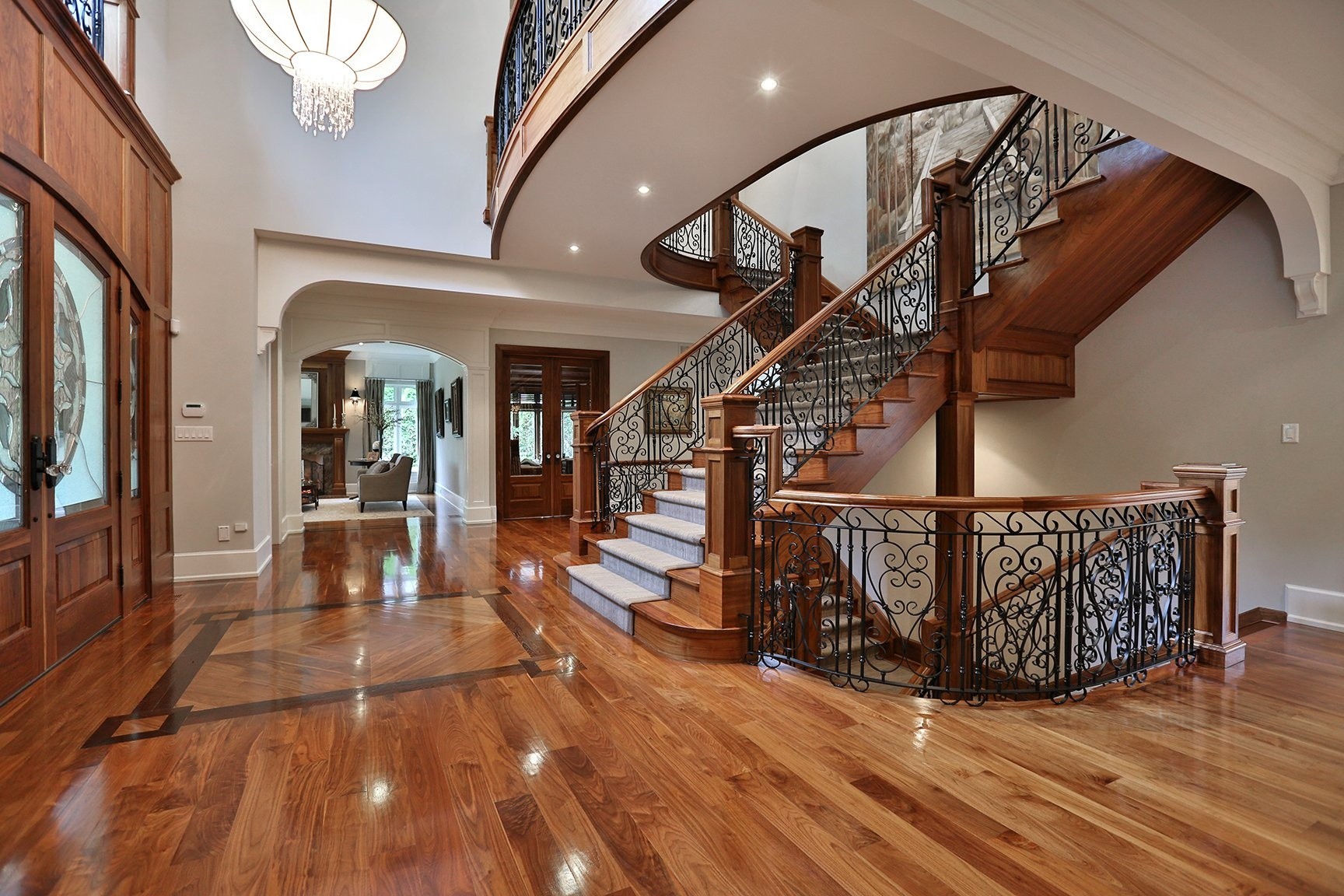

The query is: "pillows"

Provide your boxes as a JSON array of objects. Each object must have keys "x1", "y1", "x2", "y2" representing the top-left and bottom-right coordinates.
[{"x1": 367, "y1": 460, "x2": 390, "y2": 473}]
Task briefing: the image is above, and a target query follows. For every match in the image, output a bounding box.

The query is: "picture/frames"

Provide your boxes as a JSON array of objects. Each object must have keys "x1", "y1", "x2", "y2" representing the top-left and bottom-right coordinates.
[
  {"x1": 450, "y1": 376, "x2": 462, "y2": 438},
  {"x1": 644, "y1": 385, "x2": 693, "y2": 435},
  {"x1": 434, "y1": 388, "x2": 444, "y2": 440},
  {"x1": 444, "y1": 398, "x2": 451, "y2": 423}
]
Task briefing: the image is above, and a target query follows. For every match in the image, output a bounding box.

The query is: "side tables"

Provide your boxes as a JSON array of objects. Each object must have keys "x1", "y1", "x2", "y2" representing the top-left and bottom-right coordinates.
[{"x1": 347, "y1": 460, "x2": 378, "y2": 499}]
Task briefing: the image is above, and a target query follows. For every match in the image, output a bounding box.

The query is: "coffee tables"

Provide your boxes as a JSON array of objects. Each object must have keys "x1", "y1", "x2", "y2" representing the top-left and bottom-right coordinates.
[{"x1": 302, "y1": 482, "x2": 319, "y2": 510}]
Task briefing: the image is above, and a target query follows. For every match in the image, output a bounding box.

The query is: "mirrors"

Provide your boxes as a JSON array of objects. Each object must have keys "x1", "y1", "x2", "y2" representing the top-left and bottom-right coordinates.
[{"x1": 301, "y1": 372, "x2": 319, "y2": 428}]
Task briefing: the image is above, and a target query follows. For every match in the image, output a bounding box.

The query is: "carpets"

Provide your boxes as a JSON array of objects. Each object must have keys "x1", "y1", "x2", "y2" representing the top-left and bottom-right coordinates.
[{"x1": 302, "y1": 498, "x2": 434, "y2": 522}]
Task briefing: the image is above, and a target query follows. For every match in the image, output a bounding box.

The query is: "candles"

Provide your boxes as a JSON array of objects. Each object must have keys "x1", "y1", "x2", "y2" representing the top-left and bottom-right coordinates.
[
  {"x1": 334, "y1": 403, "x2": 336, "y2": 418},
  {"x1": 342, "y1": 398, "x2": 344, "y2": 413}
]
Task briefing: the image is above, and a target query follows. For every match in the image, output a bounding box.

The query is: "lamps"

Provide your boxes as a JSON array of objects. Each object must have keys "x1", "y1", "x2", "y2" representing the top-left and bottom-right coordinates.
[
  {"x1": 349, "y1": 387, "x2": 362, "y2": 404},
  {"x1": 230, "y1": 0, "x2": 408, "y2": 140}
]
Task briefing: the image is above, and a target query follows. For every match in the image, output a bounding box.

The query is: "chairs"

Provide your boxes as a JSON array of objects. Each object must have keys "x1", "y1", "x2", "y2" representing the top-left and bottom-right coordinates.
[{"x1": 357, "y1": 453, "x2": 413, "y2": 513}]
